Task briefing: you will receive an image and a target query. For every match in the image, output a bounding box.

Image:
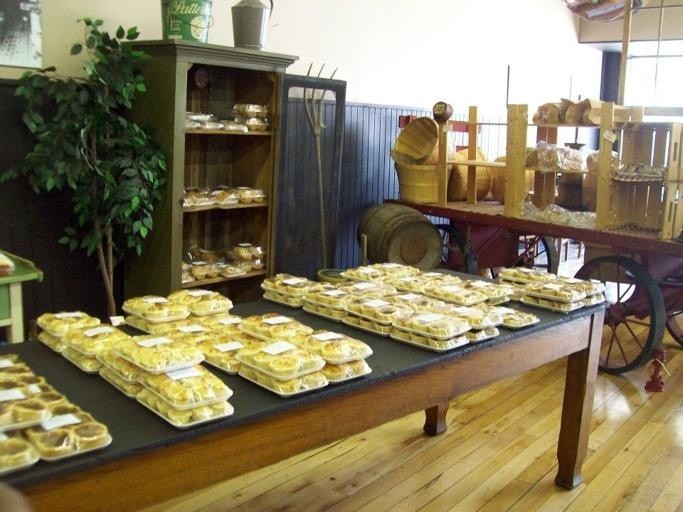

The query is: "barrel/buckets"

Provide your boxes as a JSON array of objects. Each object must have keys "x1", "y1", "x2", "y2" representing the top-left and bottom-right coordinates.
[
  {"x1": 356, "y1": 204, "x2": 442, "y2": 272},
  {"x1": 491, "y1": 157, "x2": 532, "y2": 205},
  {"x1": 390, "y1": 115, "x2": 457, "y2": 162},
  {"x1": 447, "y1": 147, "x2": 491, "y2": 201},
  {"x1": 395, "y1": 162, "x2": 453, "y2": 208}
]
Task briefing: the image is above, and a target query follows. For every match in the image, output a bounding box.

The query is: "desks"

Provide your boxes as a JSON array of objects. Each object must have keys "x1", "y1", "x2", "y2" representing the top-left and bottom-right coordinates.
[{"x1": 1, "y1": 266, "x2": 610, "y2": 509}]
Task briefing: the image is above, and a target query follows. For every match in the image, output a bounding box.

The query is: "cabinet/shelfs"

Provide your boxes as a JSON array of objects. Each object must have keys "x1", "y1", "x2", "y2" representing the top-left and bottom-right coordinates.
[{"x1": 119, "y1": 38, "x2": 299, "y2": 308}]
